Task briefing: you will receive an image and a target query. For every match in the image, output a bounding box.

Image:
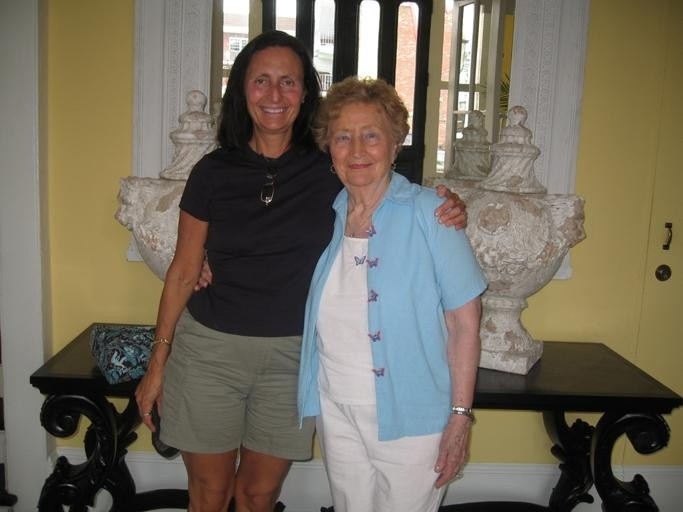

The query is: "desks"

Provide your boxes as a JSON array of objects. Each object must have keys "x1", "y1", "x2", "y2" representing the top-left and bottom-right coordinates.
[{"x1": 28, "y1": 322, "x2": 683, "y2": 512}]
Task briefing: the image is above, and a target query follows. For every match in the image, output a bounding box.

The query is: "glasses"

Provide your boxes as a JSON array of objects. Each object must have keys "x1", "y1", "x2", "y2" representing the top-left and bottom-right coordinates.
[
  {"x1": 261, "y1": 158, "x2": 275, "y2": 206},
  {"x1": 328, "y1": 128, "x2": 391, "y2": 146}
]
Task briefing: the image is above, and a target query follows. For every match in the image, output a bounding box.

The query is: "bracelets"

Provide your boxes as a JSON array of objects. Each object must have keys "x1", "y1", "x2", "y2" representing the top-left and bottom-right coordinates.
[
  {"x1": 150, "y1": 340, "x2": 170, "y2": 349},
  {"x1": 451, "y1": 406, "x2": 476, "y2": 422}
]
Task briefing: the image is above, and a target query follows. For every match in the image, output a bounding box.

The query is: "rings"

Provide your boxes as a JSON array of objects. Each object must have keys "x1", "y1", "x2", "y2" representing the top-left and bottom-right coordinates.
[{"x1": 144, "y1": 411, "x2": 152, "y2": 416}]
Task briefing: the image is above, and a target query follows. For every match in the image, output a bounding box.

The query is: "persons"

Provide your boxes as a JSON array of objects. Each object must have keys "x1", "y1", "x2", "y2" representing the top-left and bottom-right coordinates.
[
  {"x1": 297, "y1": 73, "x2": 489, "y2": 511},
  {"x1": 136, "y1": 31, "x2": 468, "y2": 511}
]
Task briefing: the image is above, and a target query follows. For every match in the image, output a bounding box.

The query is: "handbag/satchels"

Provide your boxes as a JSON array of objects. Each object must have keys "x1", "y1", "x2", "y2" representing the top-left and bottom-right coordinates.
[{"x1": 90, "y1": 325, "x2": 155, "y2": 384}]
